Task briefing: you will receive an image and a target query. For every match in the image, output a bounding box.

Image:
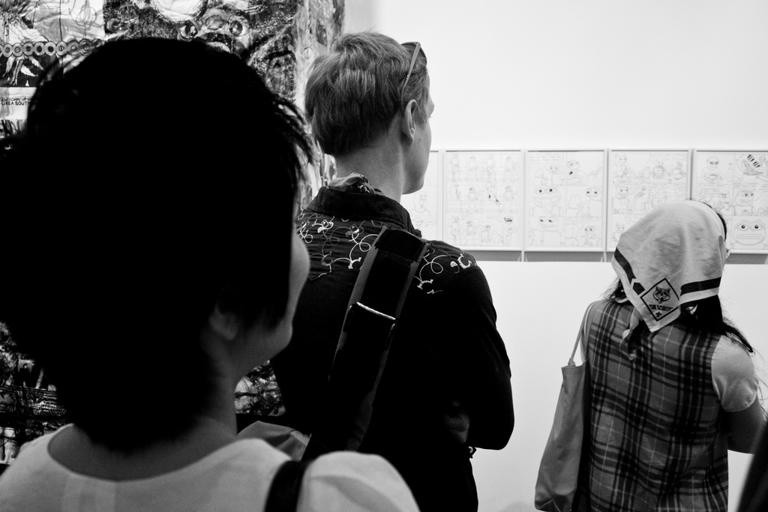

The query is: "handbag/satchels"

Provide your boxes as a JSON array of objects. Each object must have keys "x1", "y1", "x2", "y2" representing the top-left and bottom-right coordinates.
[{"x1": 536, "y1": 362, "x2": 588, "y2": 512}]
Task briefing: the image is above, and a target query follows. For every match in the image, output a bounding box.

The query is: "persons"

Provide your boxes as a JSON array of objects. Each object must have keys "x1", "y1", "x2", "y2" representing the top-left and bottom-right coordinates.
[
  {"x1": 565, "y1": 201, "x2": 767, "y2": 512},
  {"x1": 268, "y1": 30, "x2": 515, "y2": 512},
  {"x1": 0, "y1": 40, "x2": 420, "y2": 512}
]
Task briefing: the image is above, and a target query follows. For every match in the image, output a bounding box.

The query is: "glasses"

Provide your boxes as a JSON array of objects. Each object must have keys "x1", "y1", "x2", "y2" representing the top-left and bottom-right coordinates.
[{"x1": 399, "y1": 43, "x2": 427, "y2": 105}]
